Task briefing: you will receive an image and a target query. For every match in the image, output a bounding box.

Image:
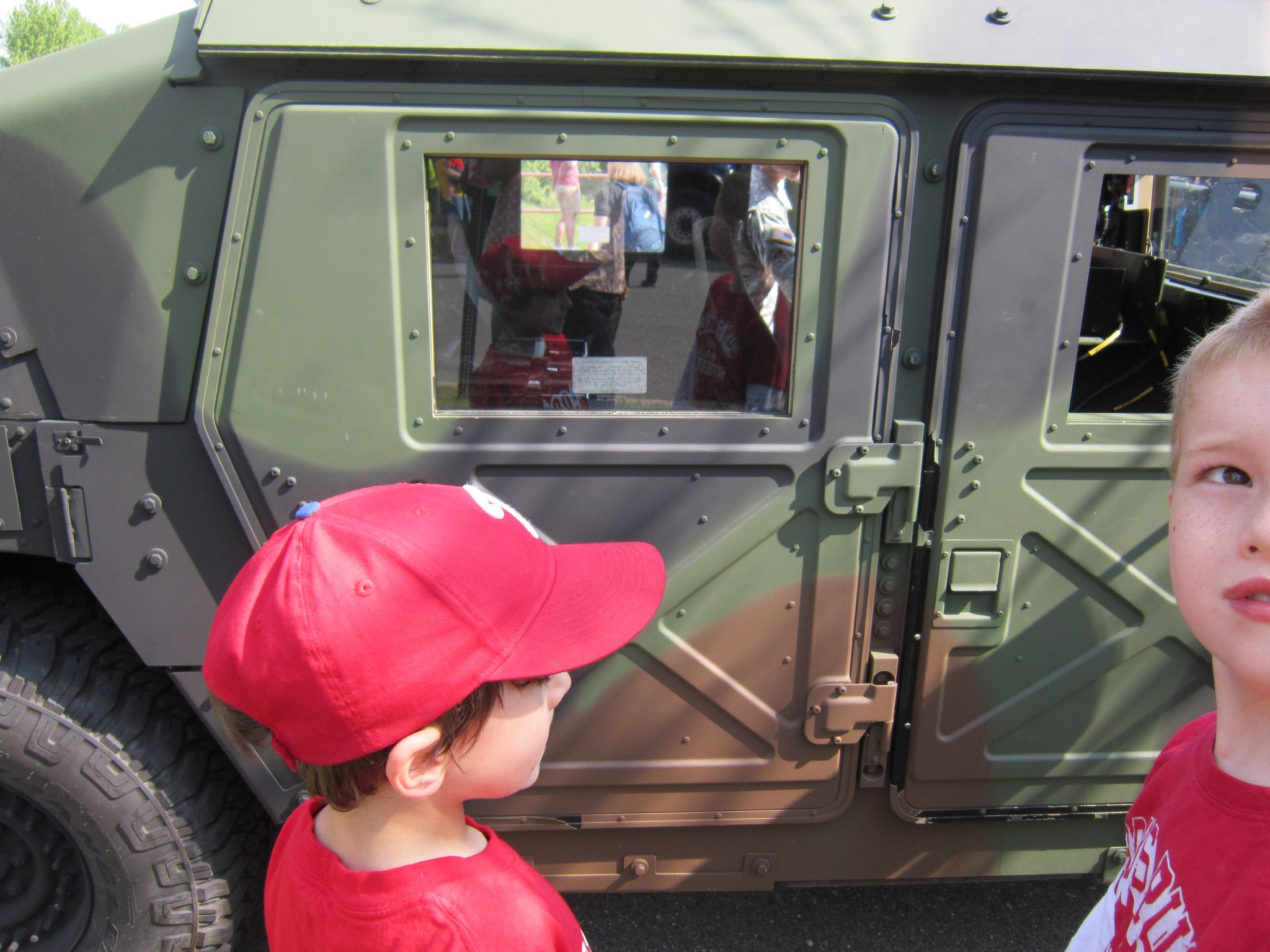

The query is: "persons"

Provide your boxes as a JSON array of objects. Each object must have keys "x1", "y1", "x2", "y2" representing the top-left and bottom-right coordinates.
[
  {"x1": 1064, "y1": 286, "x2": 1269, "y2": 951},
  {"x1": 710, "y1": 169, "x2": 800, "y2": 282},
  {"x1": 593, "y1": 160, "x2": 666, "y2": 251},
  {"x1": 445, "y1": 157, "x2": 464, "y2": 203},
  {"x1": 204, "y1": 480, "x2": 664, "y2": 952},
  {"x1": 468, "y1": 235, "x2": 598, "y2": 411},
  {"x1": 626, "y1": 252, "x2": 660, "y2": 287},
  {"x1": 436, "y1": 157, "x2": 466, "y2": 260},
  {"x1": 469, "y1": 158, "x2": 625, "y2": 410},
  {"x1": 669, "y1": 208, "x2": 792, "y2": 414},
  {"x1": 550, "y1": 161, "x2": 584, "y2": 249},
  {"x1": 747, "y1": 163, "x2": 801, "y2": 303}
]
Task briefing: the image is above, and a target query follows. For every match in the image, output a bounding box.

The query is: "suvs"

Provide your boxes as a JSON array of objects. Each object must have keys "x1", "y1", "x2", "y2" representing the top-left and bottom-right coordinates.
[{"x1": 2, "y1": 3, "x2": 1270, "y2": 952}]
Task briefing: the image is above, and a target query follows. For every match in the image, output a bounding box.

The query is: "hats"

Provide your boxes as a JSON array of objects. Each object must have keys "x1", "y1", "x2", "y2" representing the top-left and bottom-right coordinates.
[
  {"x1": 200, "y1": 482, "x2": 665, "y2": 773},
  {"x1": 480, "y1": 235, "x2": 602, "y2": 304}
]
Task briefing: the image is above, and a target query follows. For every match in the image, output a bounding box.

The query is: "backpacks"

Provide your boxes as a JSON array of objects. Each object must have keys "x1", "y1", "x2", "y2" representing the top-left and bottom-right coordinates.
[{"x1": 612, "y1": 180, "x2": 666, "y2": 254}]
